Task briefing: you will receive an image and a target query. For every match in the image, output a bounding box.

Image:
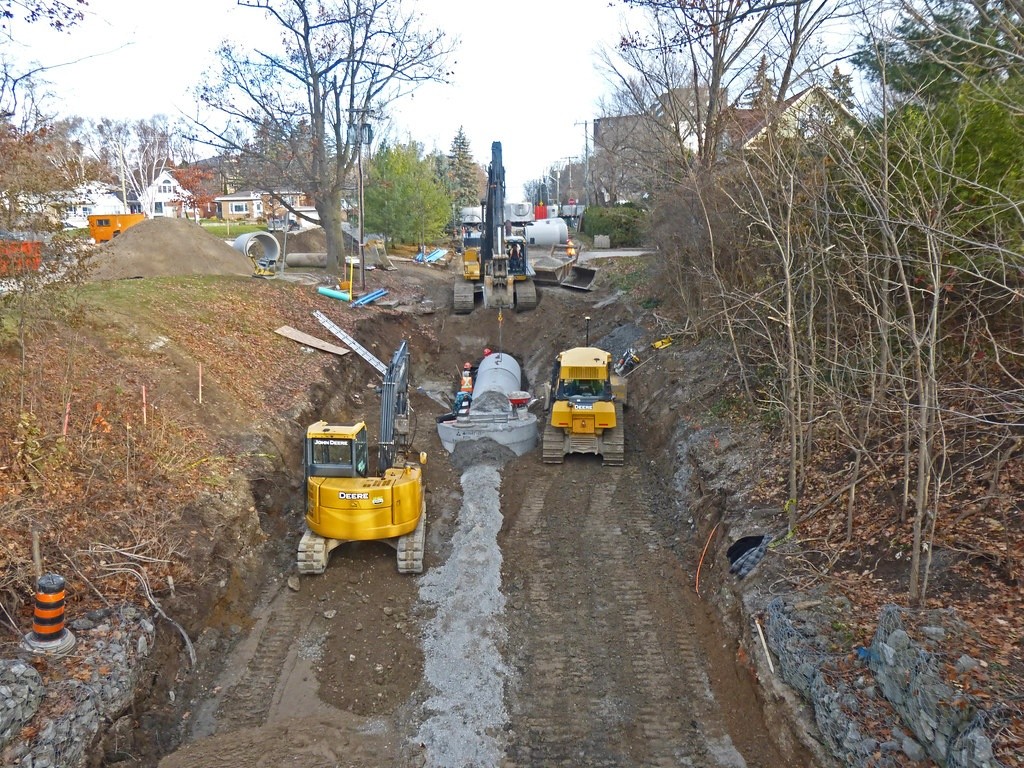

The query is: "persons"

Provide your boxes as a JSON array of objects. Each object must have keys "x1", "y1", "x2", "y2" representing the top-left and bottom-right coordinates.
[
  {"x1": 462, "y1": 362, "x2": 478, "y2": 379},
  {"x1": 509, "y1": 245, "x2": 522, "y2": 272},
  {"x1": 484, "y1": 349, "x2": 491, "y2": 355},
  {"x1": 566, "y1": 239, "x2": 576, "y2": 258}
]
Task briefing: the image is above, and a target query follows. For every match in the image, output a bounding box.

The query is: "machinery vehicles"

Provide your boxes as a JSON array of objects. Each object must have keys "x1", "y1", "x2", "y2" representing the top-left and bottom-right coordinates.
[
  {"x1": 453, "y1": 140, "x2": 536, "y2": 313},
  {"x1": 544, "y1": 316, "x2": 628, "y2": 467},
  {"x1": 297, "y1": 341, "x2": 428, "y2": 574}
]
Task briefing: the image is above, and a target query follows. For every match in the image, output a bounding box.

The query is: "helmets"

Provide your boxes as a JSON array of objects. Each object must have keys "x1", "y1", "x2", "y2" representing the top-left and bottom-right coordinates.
[
  {"x1": 566, "y1": 239, "x2": 570, "y2": 242},
  {"x1": 484, "y1": 348, "x2": 491, "y2": 355},
  {"x1": 464, "y1": 363, "x2": 472, "y2": 368}
]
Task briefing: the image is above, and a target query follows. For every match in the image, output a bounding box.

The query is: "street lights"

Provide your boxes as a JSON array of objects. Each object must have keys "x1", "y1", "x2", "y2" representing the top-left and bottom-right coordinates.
[{"x1": 344, "y1": 108, "x2": 373, "y2": 290}]
